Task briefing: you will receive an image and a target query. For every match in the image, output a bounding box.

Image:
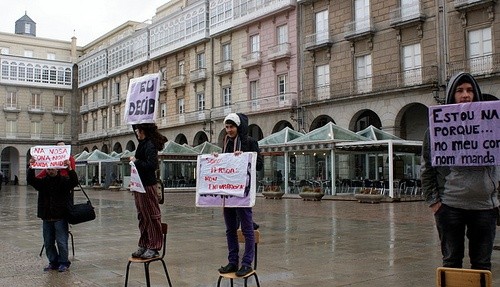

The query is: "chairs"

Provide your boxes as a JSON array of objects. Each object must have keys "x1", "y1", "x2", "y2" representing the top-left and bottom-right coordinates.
[
  {"x1": 216, "y1": 230, "x2": 260, "y2": 287},
  {"x1": 124, "y1": 223, "x2": 172, "y2": 287},
  {"x1": 437, "y1": 266, "x2": 493, "y2": 287},
  {"x1": 257, "y1": 178, "x2": 423, "y2": 201}
]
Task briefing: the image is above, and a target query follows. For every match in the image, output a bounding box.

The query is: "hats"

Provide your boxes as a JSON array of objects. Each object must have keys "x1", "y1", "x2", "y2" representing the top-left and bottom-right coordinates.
[{"x1": 223, "y1": 113, "x2": 241, "y2": 126}]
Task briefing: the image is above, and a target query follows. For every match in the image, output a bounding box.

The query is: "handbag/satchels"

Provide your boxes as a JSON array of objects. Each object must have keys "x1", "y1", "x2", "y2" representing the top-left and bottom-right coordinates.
[{"x1": 60, "y1": 182, "x2": 96, "y2": 225}]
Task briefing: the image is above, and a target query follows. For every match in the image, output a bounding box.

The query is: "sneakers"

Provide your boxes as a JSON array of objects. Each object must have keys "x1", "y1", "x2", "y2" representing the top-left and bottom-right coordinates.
[
  {"x1": 141, "y1": 249, "x2": 159, "y2": 259},
  {"x1": 58, "y1": 264, "x2": 70, "y2": 272},
  {"x1": 132, "y1": 247, "x2": 146, "y2": 257},
  {"x1": 43, "y1": 265, "x2": 59, "y2": 271}
]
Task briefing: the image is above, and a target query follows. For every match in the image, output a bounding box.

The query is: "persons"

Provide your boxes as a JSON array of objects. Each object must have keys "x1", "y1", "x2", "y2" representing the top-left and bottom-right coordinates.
[
  {"x1": 129, "y1": 123, "x2": 167, "y2": 259},
  {"x1": 26, "y1": 156, "x2": 78, "y2": 273},
  {"x1": 35, "y1": 141, "x2": 76, "y2": 178},
  {"x1": 92, "y1": 174, "x2": 98, "y2": 183},
  {"x1": 14, "y1": 175, "x2": 18, "y2": 185},
  {"x1": 178, "y1": 174, "x2": 185, "y2": 184},
  {"x1": 421, "y1": 71, "x2": 500, "y2": 274},
  {"x1": 4, "y1": 175, "x2": 9, "y2": 186},
  {"x1": 211, "y1": 113, "x2": 263, "y2": 277}
]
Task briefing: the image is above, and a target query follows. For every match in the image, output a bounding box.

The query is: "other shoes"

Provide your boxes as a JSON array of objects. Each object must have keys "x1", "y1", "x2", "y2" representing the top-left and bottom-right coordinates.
[
  {"x1": 235, "y1": 265, "x2": 252, "y2": 276},
  {"x1": 218, "y1": 263, "x2": 238, "y2": 273}
]
